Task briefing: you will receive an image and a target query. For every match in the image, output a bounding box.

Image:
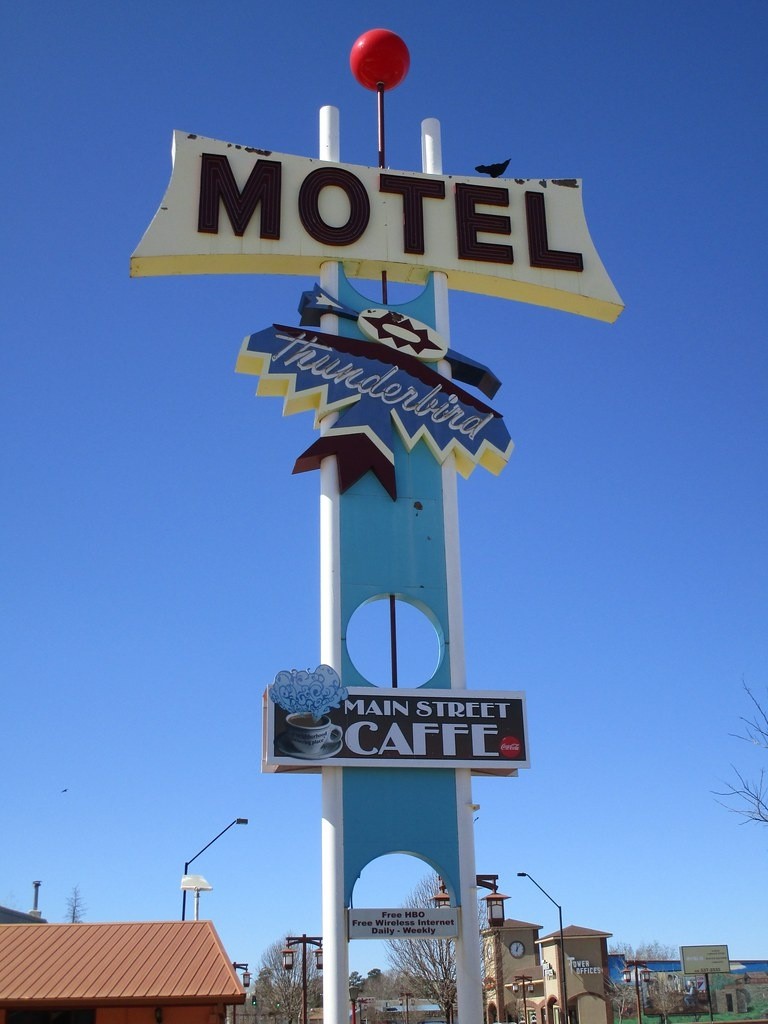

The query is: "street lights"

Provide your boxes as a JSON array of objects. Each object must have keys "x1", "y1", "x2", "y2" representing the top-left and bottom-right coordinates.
[
  {"x1": 518, "y1": 872, "x2": 568, "y2": 1024},
  {"x1": 349, "y1": 983, "x2": 359, "y2": 1024},
  {"x1": 398, "y1": 992, "x2": 415, "y2": 1024},
  {"x1": 181, "y1": 817, "x2": 249, "y2": 921},
  {"x1": 280, "y1": 934, "x2": 323, "y2": 1024},
  {"x1": 622, "y1": 960, "x2": 654, "y2": 1024},
  {"x1": 513, "y1": 974, "x2": 535, "y2": 1024},
  {"x1": 231, "y1": 962, "x2": 251, "y2": 1024}
]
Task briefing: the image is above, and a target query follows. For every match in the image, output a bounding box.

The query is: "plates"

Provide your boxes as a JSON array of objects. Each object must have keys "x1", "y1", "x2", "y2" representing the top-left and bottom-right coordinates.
[{"x1": 274, "y1": 733, "x2": 344, "y2": 759}]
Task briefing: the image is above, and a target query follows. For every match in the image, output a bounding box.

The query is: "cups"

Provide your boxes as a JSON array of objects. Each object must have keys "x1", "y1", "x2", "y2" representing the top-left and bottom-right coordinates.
[{"x1": 285, "y1": 713, "x2": 342, "y2": 752}]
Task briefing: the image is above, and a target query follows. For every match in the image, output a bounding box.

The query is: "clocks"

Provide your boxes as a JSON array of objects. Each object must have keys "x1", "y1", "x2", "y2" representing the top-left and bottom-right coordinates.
[{"x1": 510, "y1": 941, "x2": 525, "y2": 960}]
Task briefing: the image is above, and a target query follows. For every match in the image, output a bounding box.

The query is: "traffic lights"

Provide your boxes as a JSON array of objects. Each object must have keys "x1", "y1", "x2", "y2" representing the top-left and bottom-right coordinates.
[
  {"x1": 251, "y1": 995, "x2": 257, "y2": 1006},
  {"x1": 276, "y1": 1003, "x2": 280, "y2": 1008}
]
